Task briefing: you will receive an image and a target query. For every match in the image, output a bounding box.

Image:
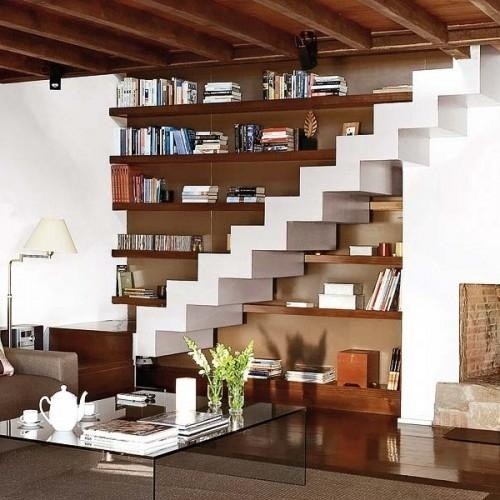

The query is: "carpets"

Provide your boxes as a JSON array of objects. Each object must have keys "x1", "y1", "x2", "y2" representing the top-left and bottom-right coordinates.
[{"x1": 1, "y1": 444, "x2": 488, "y2": 500}]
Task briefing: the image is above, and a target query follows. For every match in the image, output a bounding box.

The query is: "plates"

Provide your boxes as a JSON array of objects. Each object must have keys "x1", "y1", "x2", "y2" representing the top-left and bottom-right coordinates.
[{"x1": 17, "y1": 420, "x2": 39, "y2": 426}]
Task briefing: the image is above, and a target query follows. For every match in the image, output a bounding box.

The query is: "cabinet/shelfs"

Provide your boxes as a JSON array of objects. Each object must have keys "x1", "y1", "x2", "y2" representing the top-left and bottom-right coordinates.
[{"x1": 107, "y1": 95, "x2": 410, "y2": 420}]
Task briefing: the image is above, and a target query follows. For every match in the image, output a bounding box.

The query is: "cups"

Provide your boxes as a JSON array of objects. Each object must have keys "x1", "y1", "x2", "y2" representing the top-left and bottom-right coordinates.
[
  {"x1": 20, "y1": 410, "x2": 39, "y2": 423},
  {"x1": 85, "y1": 402, "x2": 94, "y2": 416},
  {"x1": 20, "y1": 430, "x2": 37, "y2": 440}
]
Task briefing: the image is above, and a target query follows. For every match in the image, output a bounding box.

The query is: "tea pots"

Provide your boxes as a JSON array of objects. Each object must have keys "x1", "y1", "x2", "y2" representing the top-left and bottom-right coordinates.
[{"x1": 38, "y1": 385, "x2": 88, "y2": 435}]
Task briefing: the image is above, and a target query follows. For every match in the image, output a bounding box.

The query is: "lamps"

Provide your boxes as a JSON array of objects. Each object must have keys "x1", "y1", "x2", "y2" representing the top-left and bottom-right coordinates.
[{"x1": 3, "y1": 217, "x2": 80, "y2": 348}]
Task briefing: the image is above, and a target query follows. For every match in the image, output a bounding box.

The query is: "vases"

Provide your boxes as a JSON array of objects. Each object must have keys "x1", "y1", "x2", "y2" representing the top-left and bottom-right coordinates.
[{"x1": 206, "y1": 376, "x2": 246, "y2": 416}]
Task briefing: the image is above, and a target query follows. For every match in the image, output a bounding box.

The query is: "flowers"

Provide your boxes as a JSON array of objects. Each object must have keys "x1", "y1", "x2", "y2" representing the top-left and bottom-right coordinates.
[{"x1": 184, "y1": 332, "x2": 257, "y2": 411}]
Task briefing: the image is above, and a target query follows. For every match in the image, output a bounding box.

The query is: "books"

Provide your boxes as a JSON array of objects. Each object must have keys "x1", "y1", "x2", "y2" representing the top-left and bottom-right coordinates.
[
  {"x1": 246, "y1": 357, "x2": 335, "y2": 385},
  {"x1": 234, "y1": 123, "x2": 299, "y2": 152},
  {"x1": 283, "y1": 266, "x2": 402, "y2": 311},
  {"x1": 111, "y1": 165, "x2": 168, "y2": 204},
  {"x1": 116, "y1": 264, "x2": 166, "y2": 299},
  {"x1": 83, "y1": 411, "x2": 232, "y2": 456},
  {"x1": 118, "y1": 234, "x2": 204, "y2": 251},
  {"x1": 263, "y1": 71, "x2": 347, "y2": 100},
  {"x1": 386, "y1": 347, "x2": 402, "y2": 390},
  {"x1": 202, "y1": 82, "x2": 242, "y2": 102},
  {"x1": 226, "y1": 187, "x2": 266, "y2": 202},
  {"x1": 372, "y1": 84, "x2": 413, "y2": 92},
  {"x1": 112, "y1": 116, "x2": 229, "y2": 155},
  {"x1": 182, "y1": 185, "x2": 219, "y2": 202},
  {"x1": 117, "y1": 77, "x2": 197, "y2": 108}
]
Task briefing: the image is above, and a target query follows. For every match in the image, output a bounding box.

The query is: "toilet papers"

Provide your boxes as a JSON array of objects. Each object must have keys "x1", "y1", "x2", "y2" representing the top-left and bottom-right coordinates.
[{"x1": 175, "y1": 378, "x2": 197, "y2": 424}]
